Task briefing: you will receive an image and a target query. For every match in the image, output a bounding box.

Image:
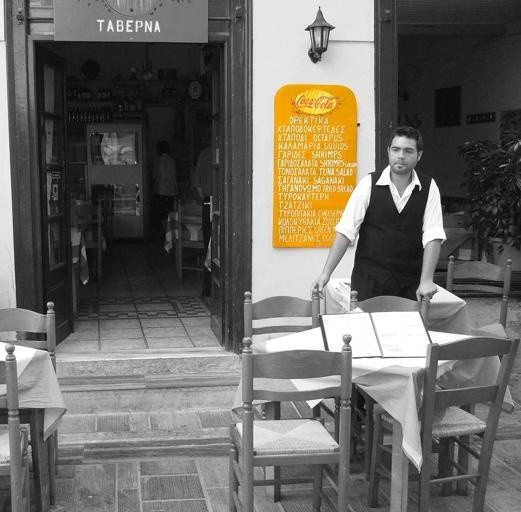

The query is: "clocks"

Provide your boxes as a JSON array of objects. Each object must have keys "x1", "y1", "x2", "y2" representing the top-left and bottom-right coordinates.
[{"x1": 187, "y1": 80, "x2": 203, "y2": 100}]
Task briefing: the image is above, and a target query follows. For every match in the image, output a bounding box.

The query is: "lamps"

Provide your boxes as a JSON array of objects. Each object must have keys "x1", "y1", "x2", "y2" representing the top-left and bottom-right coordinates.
[{"x1": 304, "y1": 7, "x2": 335, "y2": 65}]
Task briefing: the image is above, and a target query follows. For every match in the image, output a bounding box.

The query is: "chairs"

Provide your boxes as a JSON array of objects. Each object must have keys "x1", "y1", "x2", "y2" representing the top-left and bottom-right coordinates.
[
  {"x1": 0, "y1": 343, "x2": 30, "y2": 512},
  {"x1": 75, "y1": 199, "x2": 104, "y2": 285},
  {"x1": 173, "y1": 197, "x2": 205, "y2": 286},
  {"x1": 0, "y1": 301, "x2": 59, "y2": 506}
]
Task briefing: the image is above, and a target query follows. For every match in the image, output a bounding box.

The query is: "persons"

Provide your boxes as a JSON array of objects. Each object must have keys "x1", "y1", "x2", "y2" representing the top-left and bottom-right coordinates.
[
  {"x1": 192, "y1": 142, "x2": 214, "y2": 297},
  {"x1": 312, "y1": 126, "x2": 447, "y2": 425},
  {"x1": 148, "y1": 141, "x2": 179, "y2": 259}
]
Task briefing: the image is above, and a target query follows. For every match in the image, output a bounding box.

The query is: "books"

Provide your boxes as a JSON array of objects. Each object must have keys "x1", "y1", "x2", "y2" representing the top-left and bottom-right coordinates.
[{"x1": 318, "y1": 306, "x2": 434, "y2": 360}]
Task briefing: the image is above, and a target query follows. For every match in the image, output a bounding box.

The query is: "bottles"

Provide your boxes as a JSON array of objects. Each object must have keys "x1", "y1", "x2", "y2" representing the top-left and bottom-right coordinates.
[
  {"x1": 66, "y1": 79, "x2": 111, "y2": 102},
  {"x1": 111, "y1": 96, "x2": 143, "y2": 113},
  {"x1": 66, "y1": 106, "x2": 111, "y2": 129}
]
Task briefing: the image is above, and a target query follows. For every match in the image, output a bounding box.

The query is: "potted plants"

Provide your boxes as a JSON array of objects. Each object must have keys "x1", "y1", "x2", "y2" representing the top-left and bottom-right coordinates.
[{"x1": 457, "y1": 110, "x2": 521, "y2": 284}]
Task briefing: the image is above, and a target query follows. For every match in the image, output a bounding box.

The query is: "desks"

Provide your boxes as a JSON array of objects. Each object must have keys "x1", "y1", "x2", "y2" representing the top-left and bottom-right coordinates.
[{"x1": 1, "y1": 343, "x2": 67, "y2": 512}]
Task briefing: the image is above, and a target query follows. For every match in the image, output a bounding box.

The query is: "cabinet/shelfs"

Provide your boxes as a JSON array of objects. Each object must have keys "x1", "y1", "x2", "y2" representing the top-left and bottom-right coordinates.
[{"x1": 67, "y1": 81, "x2": 144, "y2": 240}]
